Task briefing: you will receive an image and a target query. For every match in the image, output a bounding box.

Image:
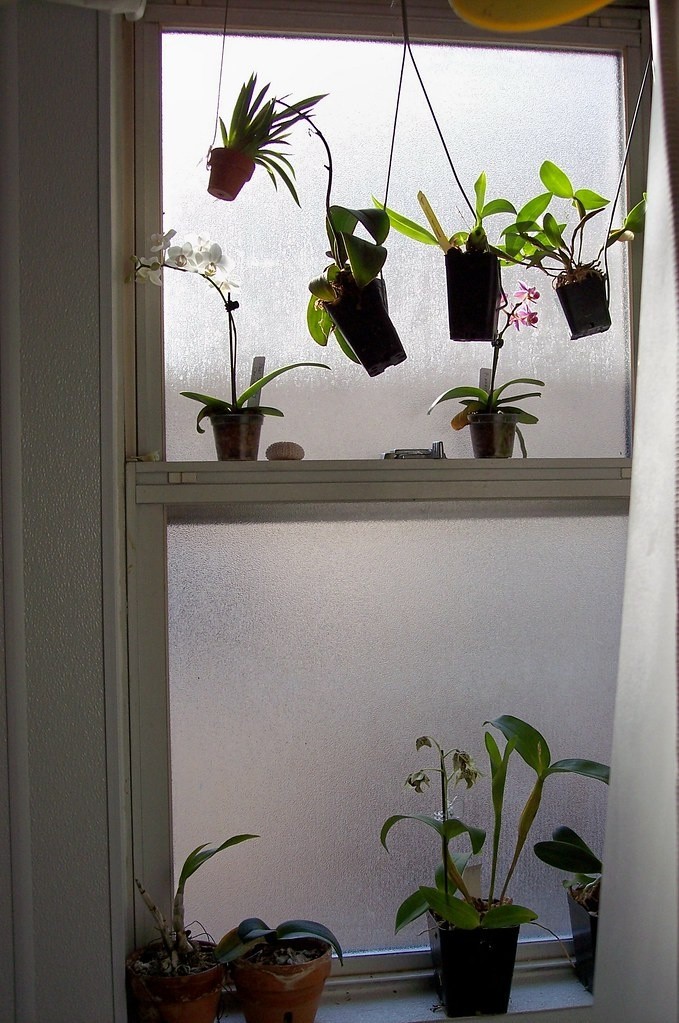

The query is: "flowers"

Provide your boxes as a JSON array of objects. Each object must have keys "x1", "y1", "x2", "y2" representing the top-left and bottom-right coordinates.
[
  {"x1": 426, "y1": 279, "x2": 546, "y2": 431},
  {"x1": 123, "y1": 228, "x2": 331, "y2": 419}
]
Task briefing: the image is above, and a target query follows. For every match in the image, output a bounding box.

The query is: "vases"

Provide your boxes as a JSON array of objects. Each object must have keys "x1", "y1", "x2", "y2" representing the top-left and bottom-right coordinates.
[
  {"x1": 466, "y1": 412, "x2": 521, "y2": 458},
  {"x1": 498, "y1": 439, "x2": 500, "y2": 440},
  {"x1": 207, "y1": 414, "x2": 265, "y2": 461}
]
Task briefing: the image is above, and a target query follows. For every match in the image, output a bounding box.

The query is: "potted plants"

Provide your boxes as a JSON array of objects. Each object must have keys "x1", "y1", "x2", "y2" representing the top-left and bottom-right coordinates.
[
  {"x1": 124, "y1": 831, "x2": 261, "y2": 1023},
  {"x1": 497, "y1": 158, "x2": 633, "y2": 339},
  {"x1": 308, "y1": 202, "x2": 409, "y2": 378},
  {"x1": 369, "y1": 713, "x2": 610, "y2": 1020},
  {"x1": 212, "y1": 917, "x2": 345, "y2": 1023},
  {"x1": 370, "y1": 170, "x2": 507, "y2": 343},
  {"x1": 532, "y1": 824, "x2": 601, "y2": 988},
  {"x1": 205, "y1": 70, "x2": 328, "y2": 208}
]
